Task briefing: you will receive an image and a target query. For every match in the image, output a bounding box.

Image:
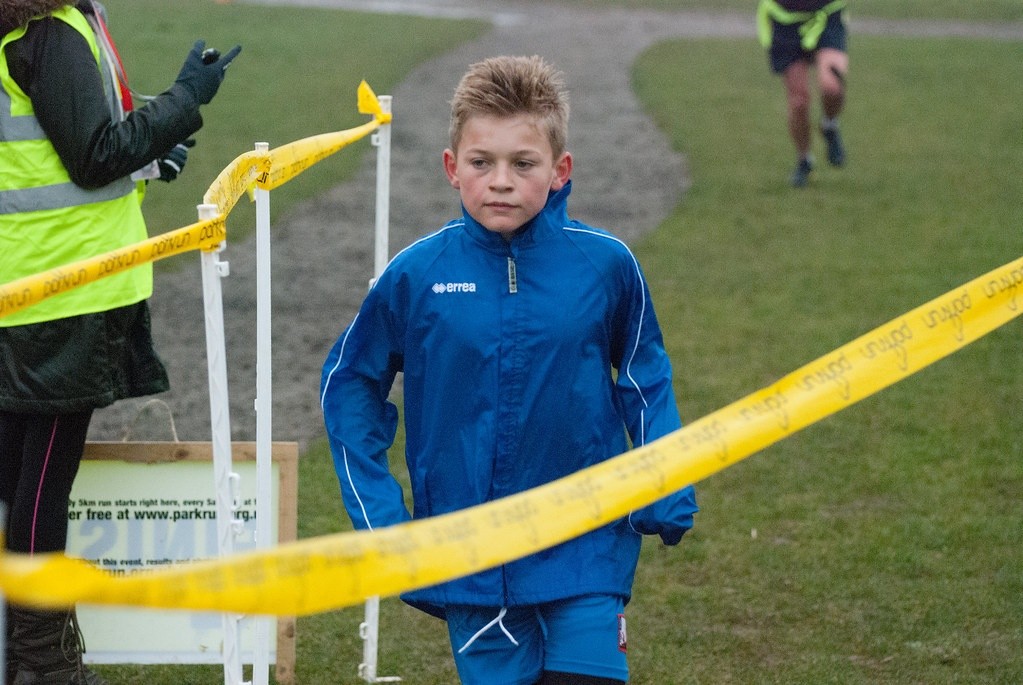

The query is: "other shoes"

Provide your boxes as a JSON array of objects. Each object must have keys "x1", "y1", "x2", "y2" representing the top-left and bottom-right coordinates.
[
  {"x1": 788, "y1": 156, "x2": 815, "y2": 190},
  {"x1": 818, "y1": 122, "x2": 846, "y2": 168}
]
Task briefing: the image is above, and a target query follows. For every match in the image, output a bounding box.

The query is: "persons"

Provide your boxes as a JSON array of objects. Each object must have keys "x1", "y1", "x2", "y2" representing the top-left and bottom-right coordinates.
[
  {"x1": 0, "y1": 1, "x2": 240, "y2": 685},
  {"x1": 317, "y1": 51, "x2": 700, "y2": 685},
  {"x1": 755, "y1": 0, "x2": 849, "y2": 190}
]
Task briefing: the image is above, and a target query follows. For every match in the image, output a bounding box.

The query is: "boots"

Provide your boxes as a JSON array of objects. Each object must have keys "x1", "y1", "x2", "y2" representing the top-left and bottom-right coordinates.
[{"x1": 5, "y1": 604, "x2": 108, "y2": 685}]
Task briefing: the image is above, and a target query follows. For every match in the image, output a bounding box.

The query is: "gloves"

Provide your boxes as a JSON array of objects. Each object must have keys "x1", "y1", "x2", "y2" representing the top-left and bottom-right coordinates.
[
  {"x1": 156, "y1": 139, "x2": 197, "y2": 182},
  {"x1": 174, "y1": 39, "x2": 242, "y2": 106}
]
